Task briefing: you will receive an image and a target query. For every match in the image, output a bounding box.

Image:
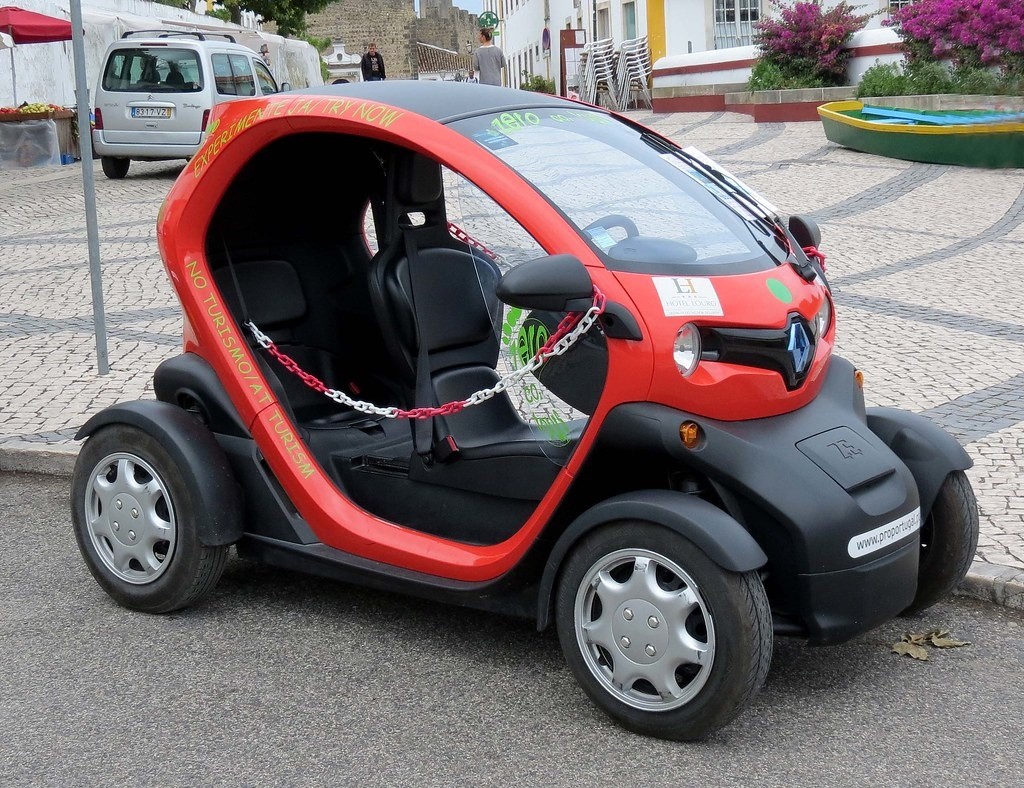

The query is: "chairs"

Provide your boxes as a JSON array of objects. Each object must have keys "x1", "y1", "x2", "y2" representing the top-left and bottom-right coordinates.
[
  {"x1": 368, "y1": 234, "x2": 589, "y2": 499},
  {"x1": 577, "y1": 36, "x2": 653, "y2": 112},
  {"x1": 212, "y1": 150, "x2": 415, "y2": 485},
  {"x1": 137, "y1": 70, "x2": 161, "y2": 87},
  {"x1": 165, "y1": 71, "x2": 200, "y2": 90}
]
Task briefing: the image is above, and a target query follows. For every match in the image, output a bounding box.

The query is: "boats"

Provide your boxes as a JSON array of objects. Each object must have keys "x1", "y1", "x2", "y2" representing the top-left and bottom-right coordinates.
[{"x1": 814, "y1": 98, "x2": 1024, "y2": 169}]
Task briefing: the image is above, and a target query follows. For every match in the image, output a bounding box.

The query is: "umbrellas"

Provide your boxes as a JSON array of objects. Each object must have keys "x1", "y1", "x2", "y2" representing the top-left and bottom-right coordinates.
[{"x1": 0, "y1": 6, "x2": 85, "y2": 108}]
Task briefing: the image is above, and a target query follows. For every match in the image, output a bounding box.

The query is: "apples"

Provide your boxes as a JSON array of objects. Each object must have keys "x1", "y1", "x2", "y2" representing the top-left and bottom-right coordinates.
[{"x1": 0, "y1": 103, "x2": 62, "y2": 114}]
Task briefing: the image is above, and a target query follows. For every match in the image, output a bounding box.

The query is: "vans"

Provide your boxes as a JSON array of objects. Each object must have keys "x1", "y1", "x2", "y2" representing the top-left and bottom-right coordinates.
[{"x1": 90, "y1": 29, "x2": 294, "y2": 180}]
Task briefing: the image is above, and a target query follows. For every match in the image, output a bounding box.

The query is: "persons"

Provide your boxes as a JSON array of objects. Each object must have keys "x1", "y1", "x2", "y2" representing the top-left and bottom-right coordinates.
[
  {"x1": 474, "y1": 28, "x2": 507, "y2": 86},
  {"x1": 361, "y1": 43, "x2": 386, "y2": 81},
  {"x1": 465, "y1": 70, "x2": 478, "y2": 83}
]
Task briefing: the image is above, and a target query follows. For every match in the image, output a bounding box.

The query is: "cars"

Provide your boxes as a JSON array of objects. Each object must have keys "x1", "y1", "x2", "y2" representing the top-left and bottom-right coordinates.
[{"x1": 67, "y1": 68, "x2": 979, "y2": 749}]
